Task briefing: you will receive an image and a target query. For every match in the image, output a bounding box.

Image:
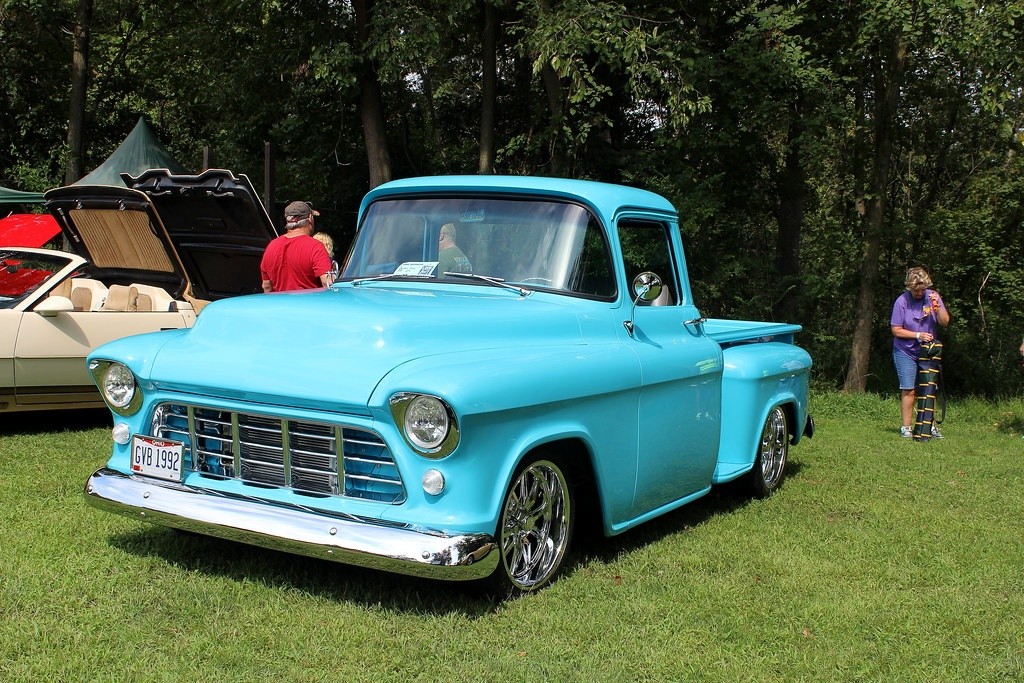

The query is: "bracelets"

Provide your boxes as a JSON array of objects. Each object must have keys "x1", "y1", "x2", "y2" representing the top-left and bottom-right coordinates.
[{"x1": 917, "y1": 332, "x2": 921, "y2": 339}]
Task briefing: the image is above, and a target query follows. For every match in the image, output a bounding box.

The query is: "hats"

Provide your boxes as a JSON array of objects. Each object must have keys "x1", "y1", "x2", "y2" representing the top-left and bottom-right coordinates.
[{"x1": 285, "y1": 201, "x2": 320, "y2": 222}]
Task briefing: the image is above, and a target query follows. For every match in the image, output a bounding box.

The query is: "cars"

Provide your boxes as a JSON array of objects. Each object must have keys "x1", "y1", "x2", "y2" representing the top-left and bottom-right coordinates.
[
  {"x1": 79, "y1": 176, "x2": 814, "y2": 593},
  {"x1": 0, "y1": 169, "x2": 284, "y2": 411}
]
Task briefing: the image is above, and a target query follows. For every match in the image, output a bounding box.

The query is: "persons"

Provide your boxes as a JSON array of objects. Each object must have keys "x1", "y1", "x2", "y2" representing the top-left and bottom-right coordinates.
[
  {"x1": 312, "y1": 232, "x2": 338, "y2": 282},
  {"x1": 891, "y1": 266, "x2": 950, "y2": 440},
  {"x1": 260, "y1": 201, "x2": 333, "y2": 293},
  {"x1": 438, "y1": 223, "x2": 473, "y2": 280},
  {"x1": 1019, "y1": 338, "x2": 1024, "y2": 356}
]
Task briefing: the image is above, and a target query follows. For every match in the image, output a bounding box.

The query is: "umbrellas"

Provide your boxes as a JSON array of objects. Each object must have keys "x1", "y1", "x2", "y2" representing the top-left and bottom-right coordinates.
[
  {"x1": 50, "y1": 117, "x2": 194, "y2": 187},
  {"x1": 0, "y1": 186, "x2": 48, "y2": 203}
]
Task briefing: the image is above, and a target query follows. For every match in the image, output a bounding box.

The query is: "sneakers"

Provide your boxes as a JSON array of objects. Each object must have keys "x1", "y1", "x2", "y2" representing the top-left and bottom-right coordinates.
[
  {"x1": 932, "y1": 428, "x2": 943, "y2": 438},
  {"x1": 901, "y1": 426, "x2": 913, "y2": 438}
]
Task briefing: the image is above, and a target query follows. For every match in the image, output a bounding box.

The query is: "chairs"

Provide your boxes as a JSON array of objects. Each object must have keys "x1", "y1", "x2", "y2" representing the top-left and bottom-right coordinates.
[{"x1": 49, "y1": 276, "x2": 152, "y2": 312}]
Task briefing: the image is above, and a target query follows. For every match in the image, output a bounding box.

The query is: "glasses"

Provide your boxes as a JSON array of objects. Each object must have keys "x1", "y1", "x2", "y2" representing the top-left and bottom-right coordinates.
[{"x1": 305, "y1": 202, "x2": 314, "y2": 215}]
